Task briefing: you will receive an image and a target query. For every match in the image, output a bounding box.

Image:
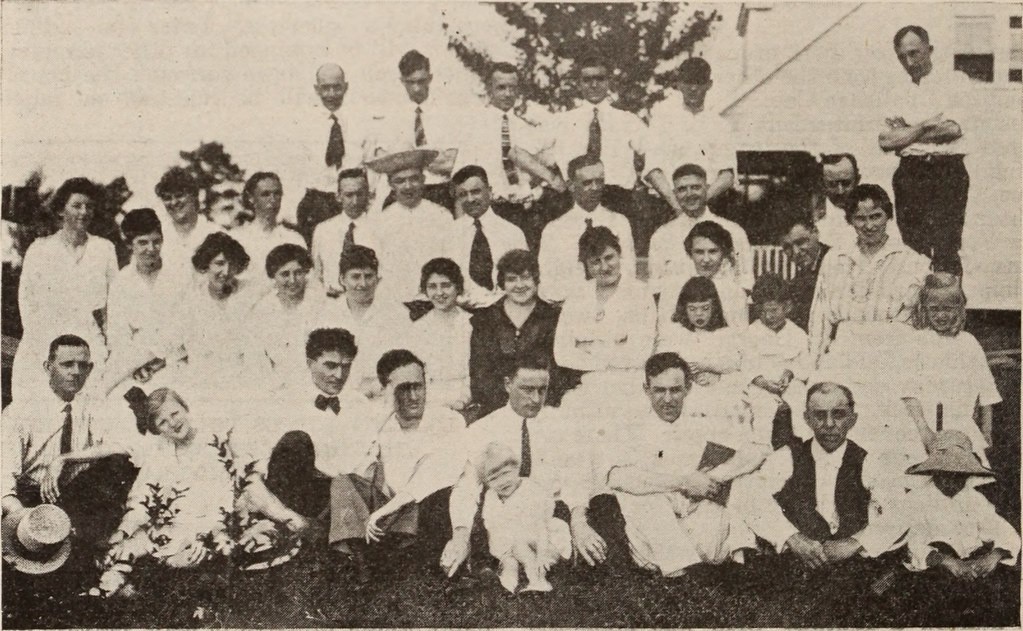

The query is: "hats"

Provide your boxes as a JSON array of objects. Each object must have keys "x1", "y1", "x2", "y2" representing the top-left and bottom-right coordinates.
[
  {"x1": 907, "y1": 429, "x2": 996, "y2": 477},
  {"x1": 2, "y1": 504, "x2": 71, "y2": 575}
]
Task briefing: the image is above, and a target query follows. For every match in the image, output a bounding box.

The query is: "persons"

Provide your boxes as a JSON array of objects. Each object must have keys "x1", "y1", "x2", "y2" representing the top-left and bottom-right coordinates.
[
  {"x1": 223, "y1": 170, "x2": 311, "y2": 297},
  {"x1": 537, "y1": 153, "x2": 637, "y2": 305},
  {"x1": 312, "y1": 143, "x2": 530, "y2": 322},
  {"x1": 740, "y1": 273, "x2": 818, "y2": 448},
  {"x1": 737, "y1": 381, "x2": 1022, "y2": 609},
  {"x1": 811, "y1": 152, "x2": 904, "y2": 248},
  {"x1": 99, "y1": 206, "x2": 194, "y2": 403},
  {"x1": 400, "y1": 257, "x2": 481, "y2": 425},
  {"x1": 552, "y1": 225, "x2": 657, "y2": 410},
  {"x1": 656, "y1": 276, "x2": 740, "y2": 385},
  {"x1": 107, "y1": 387, "x2": 308, "y2": 574},
  {"x1": 807, "y1": 183, "x2": 934, "y2": 364},
  {"x1": 171, "y1": 231, "x2": 414, "y2": 400},
  {"x1": 783, "y1": 214, "x2": 829, "y2": 334},
  {"x1": 878, "y1": 25, "x2": 970, "y2": 295},
  {"x1": 291, "y1": 51, "x2": 758, "y2": 248},
  {"x1": 647, "y1": 163, "x2": 755, "y2": 307},
  {"x1": 593, "y1": 352, "x2": 773, "y2": 586},
  {"x1": 899, "y1": 273, "x2": 1003, "y2": 498},
  {"x1": 469, "y1": 250, "x2": 575, "y2": 419},
  {"x1": 11, "y1": 179, "x2": 124, "y2": 403},
  {"x1": 155, "y1": 164, "x2": 228, "y2": 285},
  {"x1": 1, "y1": 333, "x2": 140, "y2": 612},
  {"x1": 245, "y1": 327, "x2": 622, "y2": 595}
]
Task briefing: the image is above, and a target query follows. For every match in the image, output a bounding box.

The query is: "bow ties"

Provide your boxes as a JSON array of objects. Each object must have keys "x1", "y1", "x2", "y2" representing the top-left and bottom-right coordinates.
[{"x1": 316, "y1": 395, "x2": 340, "y2": 413}]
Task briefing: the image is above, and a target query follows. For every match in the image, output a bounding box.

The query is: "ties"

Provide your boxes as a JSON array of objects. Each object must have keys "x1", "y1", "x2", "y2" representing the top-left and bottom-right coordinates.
[
  {"x1": 587, "y1": 107, "x2": 602, "y2": 159},
  {"x1": 62, "y1": 406, "x2": 73, "y2": 455},
  {"x1": 500, "y1": 115, "x2": 517, "y2": 185},
  {"x1": 469, "y1": 219, "x2": 492, "y2": 290},
  {"x1": 327, "y1": 113, "x2": 345, "y2": 169},
  {"x1": 414, "y1": 107, "x2": 428, "y2": 148},
  {"x1": 519, "y1": 419, "x2": 532, "y2": 478},
  {"x1": 342, "y1": 222, "x2": 354, "y2": 250}
]
